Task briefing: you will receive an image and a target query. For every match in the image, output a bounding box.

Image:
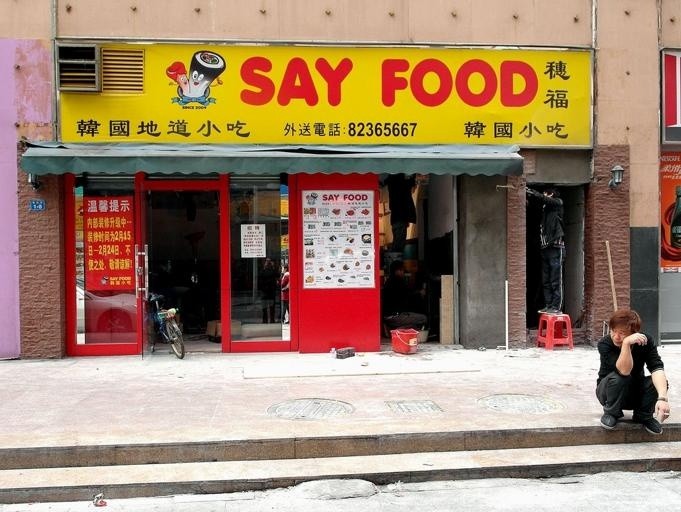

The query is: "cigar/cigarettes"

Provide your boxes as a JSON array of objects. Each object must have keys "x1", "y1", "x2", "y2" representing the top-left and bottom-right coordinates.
[{"x1": 663, "y1": 412, "x2": 671, "y2": 416}]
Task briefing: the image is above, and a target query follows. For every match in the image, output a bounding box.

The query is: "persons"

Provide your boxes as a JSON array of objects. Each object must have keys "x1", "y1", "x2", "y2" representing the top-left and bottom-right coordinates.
[
  {"x1": 379, "y1": 260, "x2": 427, "y2": 339},
  {"x1": 259, "y1": 258, "x2": 276, "y2": 323},
  {"x1": 385, "y1": 176, "x2": 420, "y2": 250},
  {"x1": 275, "y1": 263, "x2": 291, "y2": 324},
  {"x1": 524, "y1": 185, "x2": 566, "y2": 316},
  {"x1": 594, "y1": 309, "x2": 671, "y2": 436}
]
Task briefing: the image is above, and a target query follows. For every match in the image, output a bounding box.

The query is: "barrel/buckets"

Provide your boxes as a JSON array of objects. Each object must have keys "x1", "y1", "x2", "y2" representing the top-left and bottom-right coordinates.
[{"x1": 391, "y1": 329, "x2": 421, "y2": 353}]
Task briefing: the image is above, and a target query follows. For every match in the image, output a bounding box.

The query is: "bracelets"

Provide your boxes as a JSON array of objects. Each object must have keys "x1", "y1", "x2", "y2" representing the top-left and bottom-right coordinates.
[{"x1": 657, "y1": 396, "x2": 668, "y2": 402}]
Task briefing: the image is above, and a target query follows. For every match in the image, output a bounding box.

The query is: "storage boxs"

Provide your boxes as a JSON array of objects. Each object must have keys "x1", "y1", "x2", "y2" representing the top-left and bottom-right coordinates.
[{"x1": 207, "y1": 320, "x2": 242, "y2": 343}]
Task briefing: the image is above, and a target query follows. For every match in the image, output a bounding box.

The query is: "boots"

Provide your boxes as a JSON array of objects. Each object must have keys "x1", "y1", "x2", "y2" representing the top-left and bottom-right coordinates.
[{"x1": 262, "y1": 305, "x2": 276, "y2": 323}]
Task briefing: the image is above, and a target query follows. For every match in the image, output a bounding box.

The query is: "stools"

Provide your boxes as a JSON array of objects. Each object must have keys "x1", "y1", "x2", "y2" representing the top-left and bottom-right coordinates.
[{"x1": 536, "y1": 312, "x2": 574, "y2": 350}]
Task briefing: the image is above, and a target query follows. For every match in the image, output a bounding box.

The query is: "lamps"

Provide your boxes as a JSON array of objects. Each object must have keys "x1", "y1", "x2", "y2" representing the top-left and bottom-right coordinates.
[
  {"x1": 608, "y1": 165, "x2": 625, "y2": 188},
  {"x1": 28, "y1": 173, "x2": 44, "y2": 193}
]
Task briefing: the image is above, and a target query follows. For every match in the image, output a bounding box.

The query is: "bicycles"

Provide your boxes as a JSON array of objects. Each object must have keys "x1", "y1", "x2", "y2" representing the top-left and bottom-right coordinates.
[{"x1": 148, "y1": 291, "x2": 185, "y2": 359}]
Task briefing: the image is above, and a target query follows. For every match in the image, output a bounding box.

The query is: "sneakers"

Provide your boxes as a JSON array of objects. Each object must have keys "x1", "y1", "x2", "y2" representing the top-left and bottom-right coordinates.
[
  {"x1": 632, "y1": 414, "x2": 663, "y2": 435},
  {"x1": 600, "y1": 413, "x2": 624, "y2": 431},
  {"x1": 537, "y1": 306, "x2": 563, "y2": 314}
]
